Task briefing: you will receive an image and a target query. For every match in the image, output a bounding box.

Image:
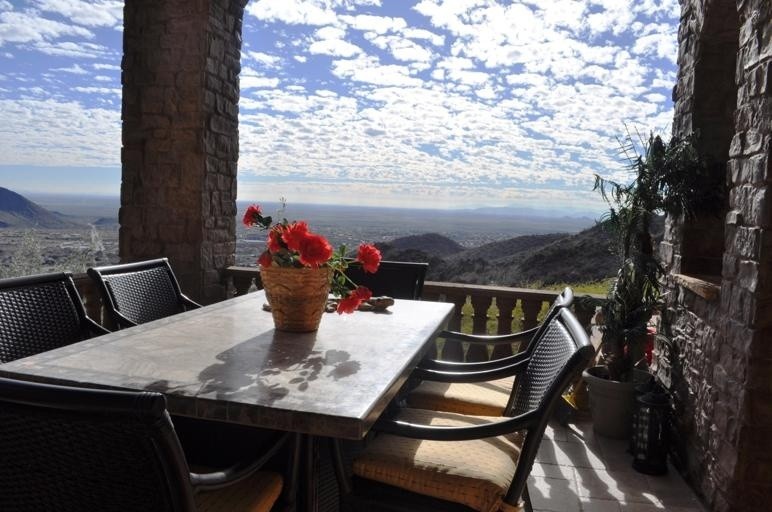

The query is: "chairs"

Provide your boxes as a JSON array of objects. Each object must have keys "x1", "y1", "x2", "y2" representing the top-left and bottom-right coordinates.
[
  {"x1": 328, "y1": 257, "x2": 429, "y2": 301},
  {"x1": 406, "y1": 285, "x2": 574, "y2": 418},
  {"x1": 329, "y1": 308, "x2": 596, "y2": 511},
  {"x1": 87, "y1": 257, "x2": 204, "y2": 332},
  {"x1": 1, "y1": 270, "x2": 114, "y2": 365},
  {"x1": 1, "y1": 377, "x2": 299, "y2": 512}
]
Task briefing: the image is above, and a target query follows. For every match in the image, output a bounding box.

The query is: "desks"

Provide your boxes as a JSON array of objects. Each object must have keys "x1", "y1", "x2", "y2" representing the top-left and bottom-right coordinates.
[{"x1": 0, "y1": 288, "x2": 455, "y2": 512}]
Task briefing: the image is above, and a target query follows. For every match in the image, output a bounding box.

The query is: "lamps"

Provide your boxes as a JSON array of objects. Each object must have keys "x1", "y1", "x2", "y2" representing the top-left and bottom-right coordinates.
[{"x1": 626, "y1": 376, "x2": 672, "y2": 474}]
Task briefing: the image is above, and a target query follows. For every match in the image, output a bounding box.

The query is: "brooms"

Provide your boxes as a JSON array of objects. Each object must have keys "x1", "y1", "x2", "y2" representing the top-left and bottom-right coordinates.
[{"x1": 555, "y1": 339, "x2": 605, "y2": 419}]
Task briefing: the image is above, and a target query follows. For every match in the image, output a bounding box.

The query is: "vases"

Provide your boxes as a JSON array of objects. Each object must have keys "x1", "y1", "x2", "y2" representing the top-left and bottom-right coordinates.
[{"x1": 259, "y1": 264, "x2": 330, "y2": 333}]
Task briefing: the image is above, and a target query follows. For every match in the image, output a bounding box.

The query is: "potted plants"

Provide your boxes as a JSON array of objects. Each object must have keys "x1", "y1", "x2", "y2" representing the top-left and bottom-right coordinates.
[{"x1": 580, "y1": 121, "x2": 710, "y2": 440}]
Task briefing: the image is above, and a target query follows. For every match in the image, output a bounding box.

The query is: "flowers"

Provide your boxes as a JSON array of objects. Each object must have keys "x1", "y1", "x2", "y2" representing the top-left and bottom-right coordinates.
[{"x1": 243, "y1": 196, "x2": 382, "y2": 315}]
